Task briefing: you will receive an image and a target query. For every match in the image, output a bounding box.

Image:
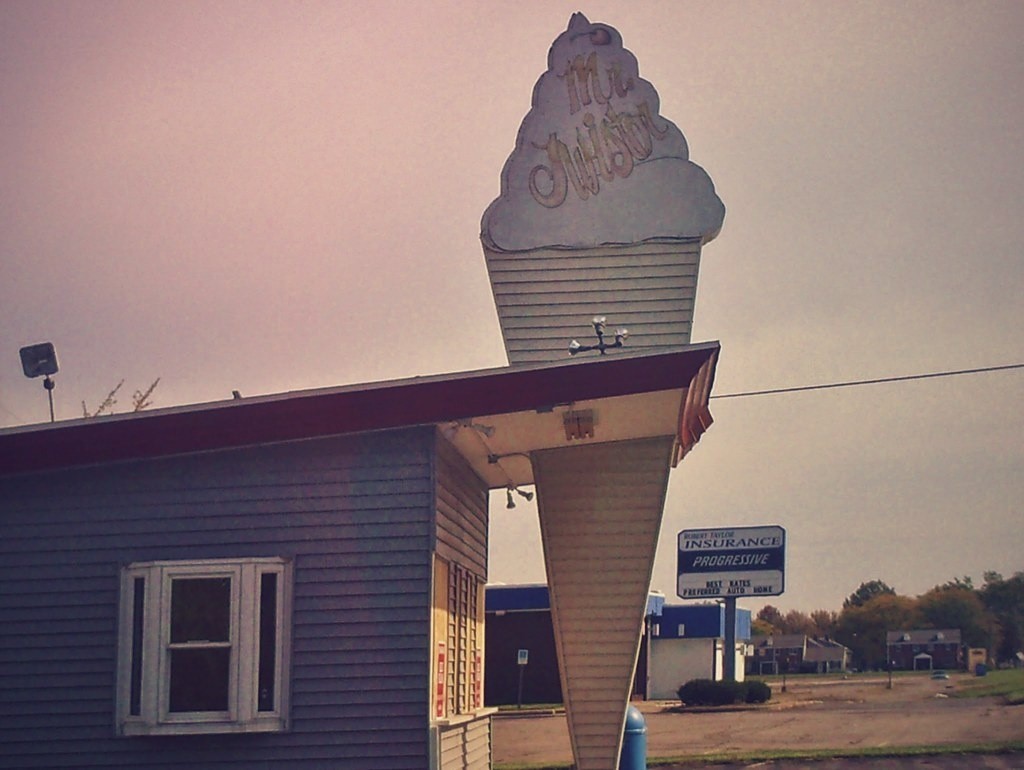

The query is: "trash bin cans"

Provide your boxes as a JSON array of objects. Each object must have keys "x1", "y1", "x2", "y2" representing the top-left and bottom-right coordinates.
[
  {"x1": 975, "y1": 664, "x2": 986, "y2": 675},
  {"x1": 620, "y1": 705, "x2": 647, "y2": 770}
]
